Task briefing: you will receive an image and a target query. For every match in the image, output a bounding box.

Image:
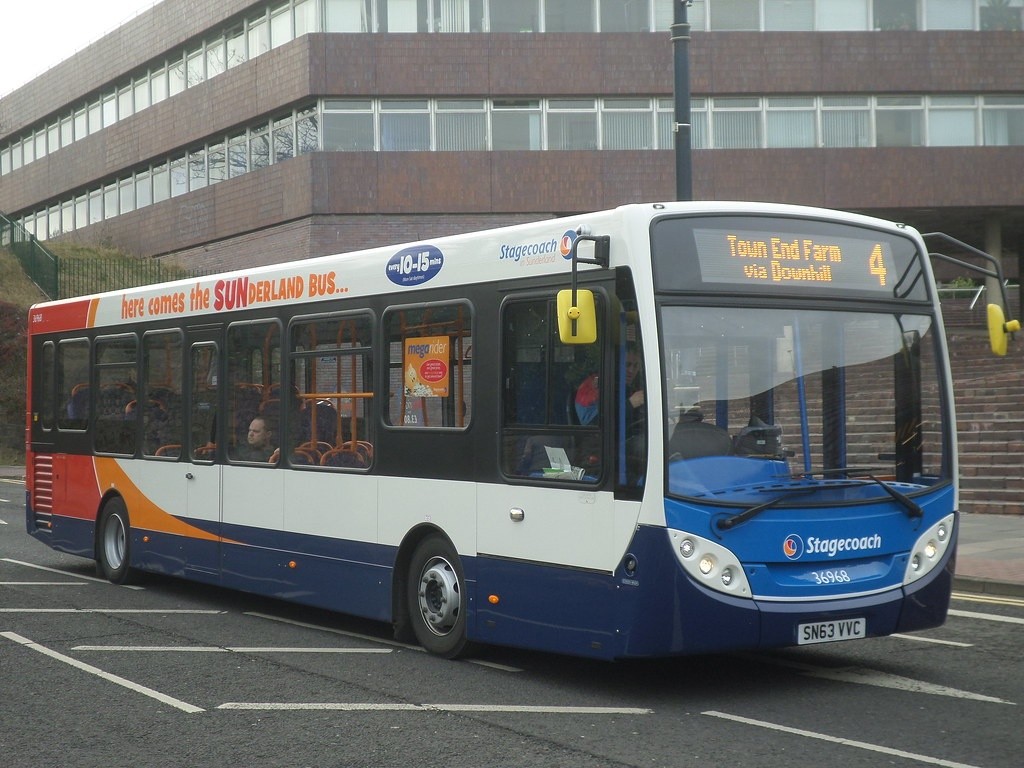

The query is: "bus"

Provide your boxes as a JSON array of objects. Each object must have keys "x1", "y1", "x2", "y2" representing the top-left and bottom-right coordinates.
[{"x1": 26, "y1": 200, "x2": 1020, "y2": 667}]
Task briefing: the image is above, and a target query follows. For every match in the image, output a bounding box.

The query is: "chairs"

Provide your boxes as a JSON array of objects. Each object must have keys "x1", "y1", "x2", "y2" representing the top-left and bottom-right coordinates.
[
  {"x1": 567, "y1": 390, "x2": 584, "y2": 457},
  {"x1": 70, "y1": 380, "x2": 372, "y2": 470}
]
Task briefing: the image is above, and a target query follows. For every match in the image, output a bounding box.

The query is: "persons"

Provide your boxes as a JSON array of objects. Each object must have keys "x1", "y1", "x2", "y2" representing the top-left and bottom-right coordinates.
[
  {"x1": 246, "y1": 418, "x2": 282, "y2": 462},
  {"x1": 574, "y1": 341, "x2": 646, "y2": 430}
]
